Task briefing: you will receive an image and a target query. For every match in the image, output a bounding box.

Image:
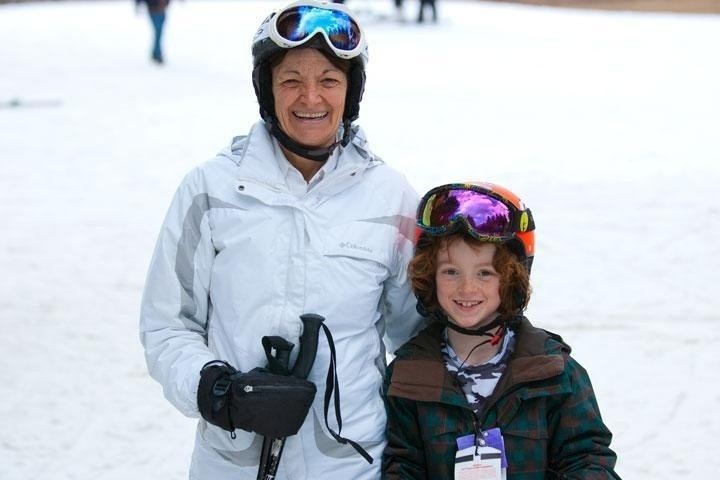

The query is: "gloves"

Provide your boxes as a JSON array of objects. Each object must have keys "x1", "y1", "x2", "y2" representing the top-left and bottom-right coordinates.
[{"x1": 195, "y1": 361, "x2": 317, "y2": 440}]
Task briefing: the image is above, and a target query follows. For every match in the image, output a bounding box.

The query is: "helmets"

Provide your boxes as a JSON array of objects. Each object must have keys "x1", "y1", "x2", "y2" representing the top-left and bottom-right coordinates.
[
  {"x1": 407, "y1": 178, "x2": 536, "y2": 311},
  {"x1": 250, "y1": 1, "x2": 366, "y2": 122}
]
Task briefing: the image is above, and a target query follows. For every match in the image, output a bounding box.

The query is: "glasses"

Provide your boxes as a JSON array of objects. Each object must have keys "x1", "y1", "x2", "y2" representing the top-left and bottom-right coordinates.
[
  {"x1": 414, "y1": 182, "x2": 540, "y2": 245},
  {"x1": 265, "y1": 0, "x2": 374, "y2": 61}
]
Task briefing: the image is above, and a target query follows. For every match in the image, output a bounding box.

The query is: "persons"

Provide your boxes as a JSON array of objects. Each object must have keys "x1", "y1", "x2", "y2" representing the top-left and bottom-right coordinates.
[
  {"x1": 134, "y1": 0, "x2": 435, "y2": 480},
  {"x1": 376, "y1": 177, "x2": 627, "y2": 479},
  {"x1": 136, "y1": 0, "x2": 175, "y2": 66}
]
